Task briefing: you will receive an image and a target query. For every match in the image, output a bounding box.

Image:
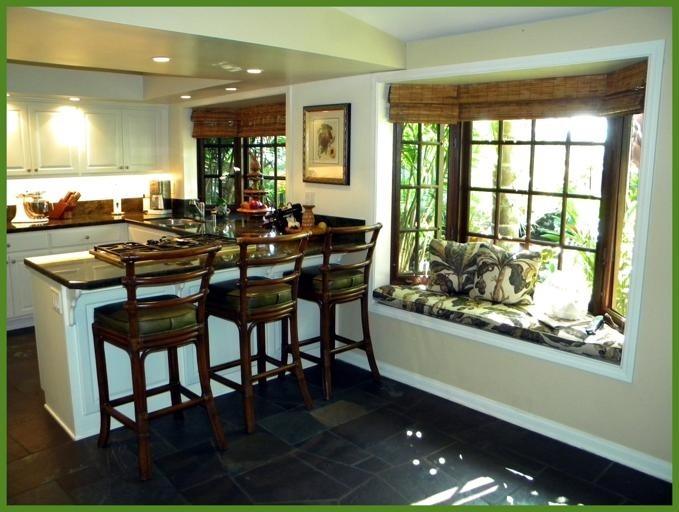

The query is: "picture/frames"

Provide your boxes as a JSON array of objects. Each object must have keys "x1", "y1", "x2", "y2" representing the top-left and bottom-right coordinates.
[{"x1": 302, "y1": 102, "x2": 351, "y2": 185}]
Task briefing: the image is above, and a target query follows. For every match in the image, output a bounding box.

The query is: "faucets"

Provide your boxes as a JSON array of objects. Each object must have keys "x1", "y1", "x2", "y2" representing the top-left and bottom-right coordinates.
[{"x1": 188, "y1": 198, "x2": 207, "y2": 220}]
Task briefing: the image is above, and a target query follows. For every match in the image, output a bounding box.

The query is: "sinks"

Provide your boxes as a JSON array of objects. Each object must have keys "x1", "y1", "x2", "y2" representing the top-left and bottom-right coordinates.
[
  {"x1": 145, "y1": 216, "x2": 203, "y2": 225},
  {"x1": 173, "y1": 223, "x2": 219, "y2": 234}
]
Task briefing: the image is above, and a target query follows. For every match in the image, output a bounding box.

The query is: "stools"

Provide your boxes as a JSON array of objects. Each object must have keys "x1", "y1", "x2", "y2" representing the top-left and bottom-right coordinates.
[
  {"x1": 87, "y1": 243, "x2": 229, "y2": 480},
  {"x1": 278, "y1": 223, "x2": 383, "y2": 401},
  {"x1": 206, "y1": 229, "x2": 315, "y2": 436}
]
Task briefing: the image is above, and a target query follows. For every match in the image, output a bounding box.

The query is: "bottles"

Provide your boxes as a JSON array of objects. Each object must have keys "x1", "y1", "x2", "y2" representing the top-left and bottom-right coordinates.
[{"x1": 303, "y1": 204, "x2": 315, "y2": 227}]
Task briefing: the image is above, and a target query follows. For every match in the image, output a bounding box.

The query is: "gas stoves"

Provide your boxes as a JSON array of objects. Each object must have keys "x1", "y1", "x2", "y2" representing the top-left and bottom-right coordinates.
[{"x1": 93, "y1": 232, "x2": 239, "y2": 261}]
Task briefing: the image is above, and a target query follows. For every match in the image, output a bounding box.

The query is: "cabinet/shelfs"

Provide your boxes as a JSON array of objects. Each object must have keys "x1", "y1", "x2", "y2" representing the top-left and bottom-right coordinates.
[
  {"x1": 182, "y1": 274, "x2": 268, "y2": 387},
  {"x1": 7, "y1": 92, "x2": 169, "y2": 179},
  {"x1": 8, "y1": 224, "x2": 129, "y2": 329},
  {"x1": 269, "y1": 258, "x2": 335, "y2": 360},
  {"x1": 66, "y1": 289, "x2": 185, "y2": 417}
]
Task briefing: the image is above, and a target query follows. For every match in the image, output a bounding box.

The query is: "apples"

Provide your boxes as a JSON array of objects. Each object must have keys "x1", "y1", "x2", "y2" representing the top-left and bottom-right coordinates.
[{"x1": 240, "y1": 200, "x2": 265, "y2": 209}]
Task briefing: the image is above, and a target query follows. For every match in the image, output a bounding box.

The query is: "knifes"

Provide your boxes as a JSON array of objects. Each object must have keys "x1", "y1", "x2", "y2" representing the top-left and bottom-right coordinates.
[{"x1": 64, "y1": 190, "x2": 81, "y2": 203}]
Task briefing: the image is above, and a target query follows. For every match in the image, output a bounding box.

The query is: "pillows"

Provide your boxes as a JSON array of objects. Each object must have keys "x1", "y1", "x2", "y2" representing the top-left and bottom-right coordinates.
[{"x1": 426, "y1": 239, "x2": 543, "y2": 305}]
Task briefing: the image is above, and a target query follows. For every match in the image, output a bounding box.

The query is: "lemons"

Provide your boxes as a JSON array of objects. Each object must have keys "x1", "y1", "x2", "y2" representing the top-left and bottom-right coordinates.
[{"x1": 319, "y1": 222, "x2": 326, "y2": 228}]
